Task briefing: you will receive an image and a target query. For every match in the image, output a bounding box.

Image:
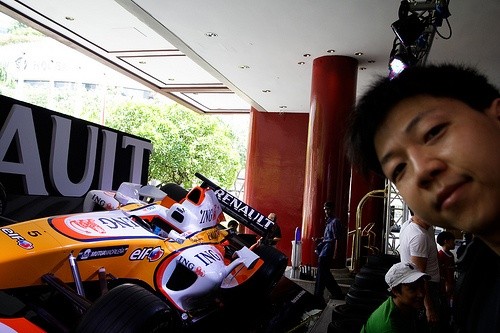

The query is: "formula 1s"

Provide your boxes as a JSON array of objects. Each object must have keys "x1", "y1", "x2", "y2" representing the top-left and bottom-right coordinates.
[{"x1": 0, "y1": 171, "x2": 317, "y2": 333}]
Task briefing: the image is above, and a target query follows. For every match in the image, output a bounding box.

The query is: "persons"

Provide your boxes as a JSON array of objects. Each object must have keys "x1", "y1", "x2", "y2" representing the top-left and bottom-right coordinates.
[
  {"x1": 360, "y1": 262, "x2": 432, "y2": 333},
  {"x1": 312, "y1": 202, "x2": 344, "y2": 309},
  {"x1": 260, "y1": 213, "x2": 282, "y2": 249},
  {"x1": 400, "y1": 205, "x2": 443, "y2": 333},
  {"x1": 347, "y1": 63, "x2": 500, "y2": 333},
  {"x1": 228, "y1": 221, "x2": 238, "y2": 234},
  {"x1": 437, "y1": 231, "x2": 474, "y2": 325}
]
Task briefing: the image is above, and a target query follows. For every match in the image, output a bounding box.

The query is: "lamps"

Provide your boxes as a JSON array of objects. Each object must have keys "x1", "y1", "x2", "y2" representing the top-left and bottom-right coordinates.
[
  {"x1": 391, "y1": 13, "x2": 428, "y2": 48},
  {"x1": 388, "y1": 51, "x2": 416, "y2": 78}
]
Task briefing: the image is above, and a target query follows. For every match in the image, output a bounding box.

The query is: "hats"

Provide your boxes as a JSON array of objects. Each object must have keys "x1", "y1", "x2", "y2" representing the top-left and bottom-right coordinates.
[
  {"x1": 385, "y1": 261, "x2": 429, "y2": 291},
  {"x1": 324, "y1": 201, "x2": 335, "y2": 208}
]
